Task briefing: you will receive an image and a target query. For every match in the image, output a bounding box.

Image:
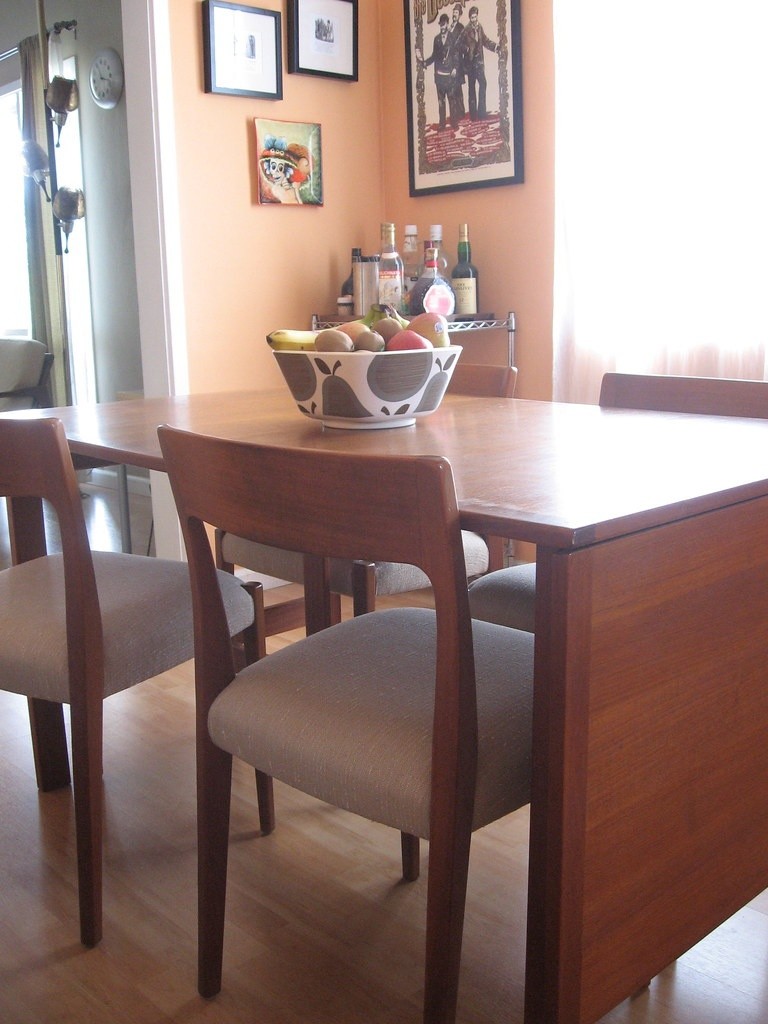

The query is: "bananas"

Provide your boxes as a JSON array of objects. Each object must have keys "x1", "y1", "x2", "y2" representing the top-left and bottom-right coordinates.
[{"x1": 266, "y1": 304, "x2": 411, "y2": 351}]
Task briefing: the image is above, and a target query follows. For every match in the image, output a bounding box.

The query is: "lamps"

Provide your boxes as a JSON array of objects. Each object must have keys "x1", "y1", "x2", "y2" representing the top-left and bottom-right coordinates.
[{"x1": 18, "y1": 75, "x2": 87, "y2": 255}]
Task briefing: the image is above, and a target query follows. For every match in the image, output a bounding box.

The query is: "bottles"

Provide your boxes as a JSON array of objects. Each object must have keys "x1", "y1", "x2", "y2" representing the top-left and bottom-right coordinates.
[
  {"x1": 451, "y1": 223, "x2": 479, "y2": 315},
  {"x1": 340, "y1": 247, "x2": 361, "y2": 297},
  {"x1": 375, "y1": 222, "x2": 405, "y2": 314},
  {"x1": 408, "y1": 225, "x2": 457, "y2": 316},
  {"x1": 401, "y1": 224, "x2": 421, "y2": 315}
]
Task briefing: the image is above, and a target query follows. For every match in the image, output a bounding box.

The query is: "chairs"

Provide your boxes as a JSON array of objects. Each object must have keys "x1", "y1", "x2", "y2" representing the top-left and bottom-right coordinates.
[
  {"x1": 156, "y1": 423, "x2": 653, "y2": 1024},
  {"x1": 469, "y1": 372, "x2": 768, "y2": 633},
  {"x1": 0, "y1": 338, "x2": 55, "y2": 412},
  {"x1": 0, "y1": 417, "x2": 276, "y2": 950},
  {"x1": 214, "y1": 363, "x2": 519, "y2": 618}
]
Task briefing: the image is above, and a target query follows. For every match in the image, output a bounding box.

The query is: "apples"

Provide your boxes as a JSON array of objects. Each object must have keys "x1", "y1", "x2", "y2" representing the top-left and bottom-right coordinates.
[
  {"x1": 385, "y1": 329, "x2": 434, "y2": 351},
  {"x1": 406, "y1": 312, "x2": 450, "y2": 348},
  {"x1": 336, "y1": 322, "x2": 370, "y2": 343}
]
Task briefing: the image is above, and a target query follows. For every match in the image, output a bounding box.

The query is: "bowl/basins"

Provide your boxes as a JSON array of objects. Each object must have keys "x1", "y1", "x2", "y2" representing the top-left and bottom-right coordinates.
[{"x1": 271, "y1": 344, "x2": 463, "y2": 429}]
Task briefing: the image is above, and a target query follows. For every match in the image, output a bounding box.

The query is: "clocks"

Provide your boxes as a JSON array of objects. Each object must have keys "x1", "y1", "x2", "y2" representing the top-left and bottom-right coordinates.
[{"x1": 88, "y1": 47, "x2": 123, "y2": 109}]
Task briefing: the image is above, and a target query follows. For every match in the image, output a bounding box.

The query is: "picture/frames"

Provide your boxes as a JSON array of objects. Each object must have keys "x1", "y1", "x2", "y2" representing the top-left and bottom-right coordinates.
[
  {"x1": 403, "y1": 0, "x2": 526, "y2": 198},
  {"x1": 286, "y1": 0, "x2": 359, "y2": 83},
  {"x1": 201, "y1": 0, "x2": 284, "y2": 102}
]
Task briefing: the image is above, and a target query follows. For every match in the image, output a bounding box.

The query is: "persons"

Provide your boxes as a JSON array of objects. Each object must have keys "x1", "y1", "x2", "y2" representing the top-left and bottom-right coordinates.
[
  {"x1": 422, "y1": 13, "x2": 458, "y2": 131},
  {"x1": 453, "y1": 6, "x2": 501, "y2": 120},
  {"x1": 450, "y1": 3, "x2": 468, "y2": 119}
]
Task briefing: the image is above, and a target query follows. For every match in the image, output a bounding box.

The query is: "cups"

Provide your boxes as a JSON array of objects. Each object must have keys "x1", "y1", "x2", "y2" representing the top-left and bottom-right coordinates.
[{"x1": 353, "y1": 256, "x2": 380, "y2": 318}]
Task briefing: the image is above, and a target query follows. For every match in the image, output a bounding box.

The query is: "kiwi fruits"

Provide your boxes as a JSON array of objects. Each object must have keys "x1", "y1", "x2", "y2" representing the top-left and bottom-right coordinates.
[{"x1": 314, "y1": 317, "x2": 404, "y2": 352}]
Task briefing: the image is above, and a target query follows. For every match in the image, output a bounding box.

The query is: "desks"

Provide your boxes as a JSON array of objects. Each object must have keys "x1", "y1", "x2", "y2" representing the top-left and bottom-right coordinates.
[{"x1": 0, "y1": 385, "x2": 768, "y2": 1024}]
